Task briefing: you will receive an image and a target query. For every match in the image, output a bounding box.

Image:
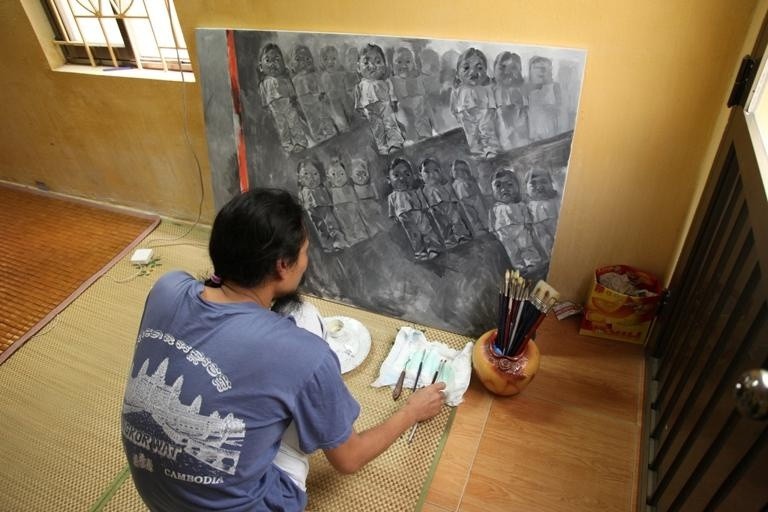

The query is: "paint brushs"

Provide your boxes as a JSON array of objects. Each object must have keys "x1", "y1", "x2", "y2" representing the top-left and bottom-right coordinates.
[
  {"x1": 413, "y1": 348, "x2": 427, "y2": 392},
  {"x1": 496, "y1": 269, "x2": 559, "y2": 356},
  {"x1": 409, "y1": 359, "x2": 447, "y2": 444}
]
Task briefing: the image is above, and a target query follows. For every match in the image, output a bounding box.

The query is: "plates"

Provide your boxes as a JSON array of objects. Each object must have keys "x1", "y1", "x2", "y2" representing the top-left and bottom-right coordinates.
[{"x1": 319, "y1": 316, "x2": 371, "y2": 376}]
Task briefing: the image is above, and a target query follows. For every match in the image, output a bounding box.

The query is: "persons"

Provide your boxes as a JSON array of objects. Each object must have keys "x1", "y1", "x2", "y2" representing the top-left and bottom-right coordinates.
[{"x1": 119, "y1": 188, "x2": 446, "y2": 512}]
